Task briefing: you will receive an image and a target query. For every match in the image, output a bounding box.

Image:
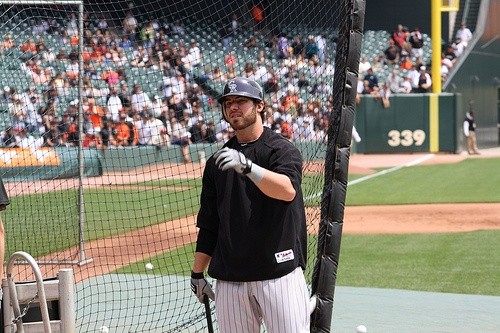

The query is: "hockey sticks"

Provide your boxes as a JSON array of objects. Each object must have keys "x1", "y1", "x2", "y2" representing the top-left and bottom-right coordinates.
[{"x1": 204, "y1": 294, "x2": 214, "y2": 333}]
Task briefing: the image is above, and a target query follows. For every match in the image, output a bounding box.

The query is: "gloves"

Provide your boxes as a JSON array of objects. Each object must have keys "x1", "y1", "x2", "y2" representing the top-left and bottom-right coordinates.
[
  {"x1": 190, "y1": 270, "x2": 215, "y2": 303},
  {"x1": 213, "y1": 147, "x2": 252, "y2": 176}
]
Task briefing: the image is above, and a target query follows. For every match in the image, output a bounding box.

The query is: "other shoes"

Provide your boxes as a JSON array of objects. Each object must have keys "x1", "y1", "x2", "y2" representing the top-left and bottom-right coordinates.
[{"x1": 469, "y1": 150, "x2": 480, "y2": 154}]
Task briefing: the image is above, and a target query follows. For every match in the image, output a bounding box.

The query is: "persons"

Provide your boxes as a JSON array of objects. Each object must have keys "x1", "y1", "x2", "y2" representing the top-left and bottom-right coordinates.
[
  {"x1": 1, "y1": 1, "x2": 482, "y2": 162},
  {"x1": 0, "y1": 179, "x2": 12, "y2": 310},
  {"x1": 191, "y1": 76, "x2": 311, "y2": 333}
]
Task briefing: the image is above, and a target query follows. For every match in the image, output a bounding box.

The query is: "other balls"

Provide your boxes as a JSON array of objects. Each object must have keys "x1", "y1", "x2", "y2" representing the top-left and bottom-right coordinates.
[
  {"x1": 356, "y1": 324, "x2": 367, "y2": 333},
  {"x1": 145, "y1": 263, "x2": 153, "y2": 269},
  {"x1": 99, "y1": 326, "x2": 108, "y2": 333}
]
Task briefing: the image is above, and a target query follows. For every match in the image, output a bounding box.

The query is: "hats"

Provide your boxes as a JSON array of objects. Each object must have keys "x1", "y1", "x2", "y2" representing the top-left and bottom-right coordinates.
[{"x1": 217, "y1": 77, "x2": 264, "y2": 102}]
